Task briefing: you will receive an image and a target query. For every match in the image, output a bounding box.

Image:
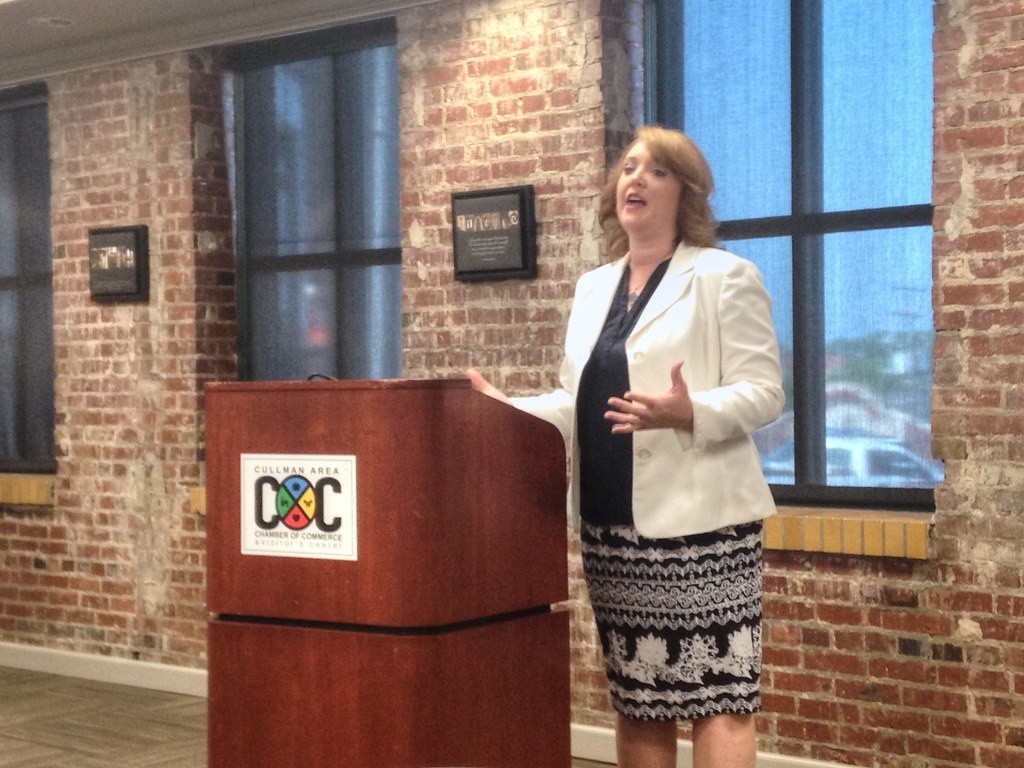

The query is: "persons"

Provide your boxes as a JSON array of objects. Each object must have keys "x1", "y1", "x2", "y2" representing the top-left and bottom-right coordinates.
[{"x1": 467, "y1": 128, "x2": 786, "y2": 768}]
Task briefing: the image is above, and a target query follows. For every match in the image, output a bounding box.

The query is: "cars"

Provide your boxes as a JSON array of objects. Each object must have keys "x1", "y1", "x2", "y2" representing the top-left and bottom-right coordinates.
[{"x1": 759, "y1": 434, "x2": 947, "y2": 488}]
